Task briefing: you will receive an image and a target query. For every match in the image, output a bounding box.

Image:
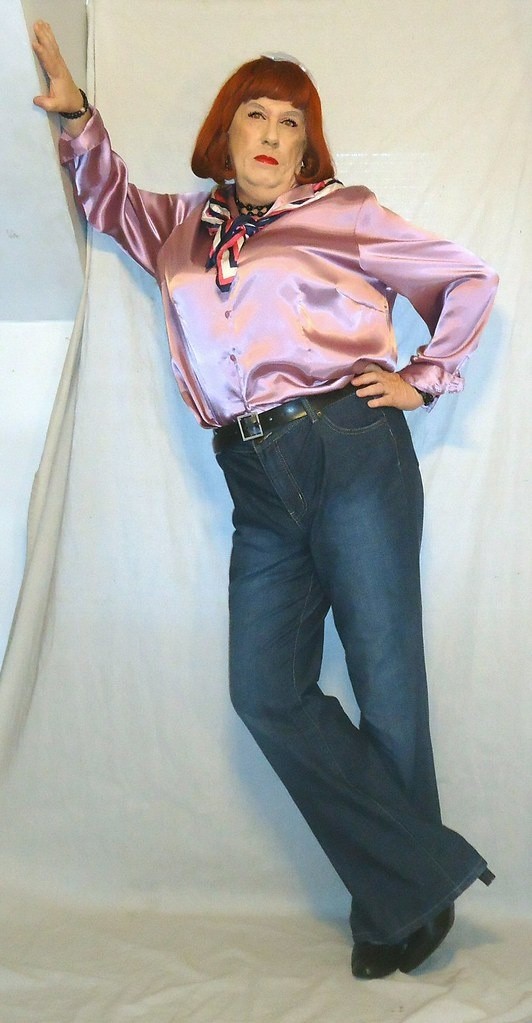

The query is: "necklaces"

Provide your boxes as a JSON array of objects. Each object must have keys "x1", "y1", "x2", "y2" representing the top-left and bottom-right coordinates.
[{"x1": 234, "y1": 196, "x2": 276, "y2": 218}]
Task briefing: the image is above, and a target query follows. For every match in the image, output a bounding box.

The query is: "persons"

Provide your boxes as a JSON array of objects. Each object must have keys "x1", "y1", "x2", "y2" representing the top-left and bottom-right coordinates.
[{"x1": 29, "y1": 17, "x2": 503, "y2": 980}]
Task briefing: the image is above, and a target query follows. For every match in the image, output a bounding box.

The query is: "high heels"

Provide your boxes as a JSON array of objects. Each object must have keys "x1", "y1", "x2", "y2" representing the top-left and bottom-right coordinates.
[
  {"x1": 351, "y1": 938, "x2": 398, "y2": 980},
  {"x1": 396, "y1": 866, "x2": 496, "y2": 973}
]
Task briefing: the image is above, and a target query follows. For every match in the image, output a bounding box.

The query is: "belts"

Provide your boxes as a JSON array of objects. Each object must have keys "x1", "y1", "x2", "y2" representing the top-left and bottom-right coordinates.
[{"x1": 211, "y1": 373, "x2": 377, "y2": 454}]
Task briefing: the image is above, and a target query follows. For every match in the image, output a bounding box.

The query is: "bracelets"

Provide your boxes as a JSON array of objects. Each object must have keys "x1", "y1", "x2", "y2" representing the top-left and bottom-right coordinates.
[
  {"x1": 58, "y1": 88, "x2": 89, "y2": 119},
  {"x1": 414, "y1": 385, "x2": 434, "y2": 406}
]
